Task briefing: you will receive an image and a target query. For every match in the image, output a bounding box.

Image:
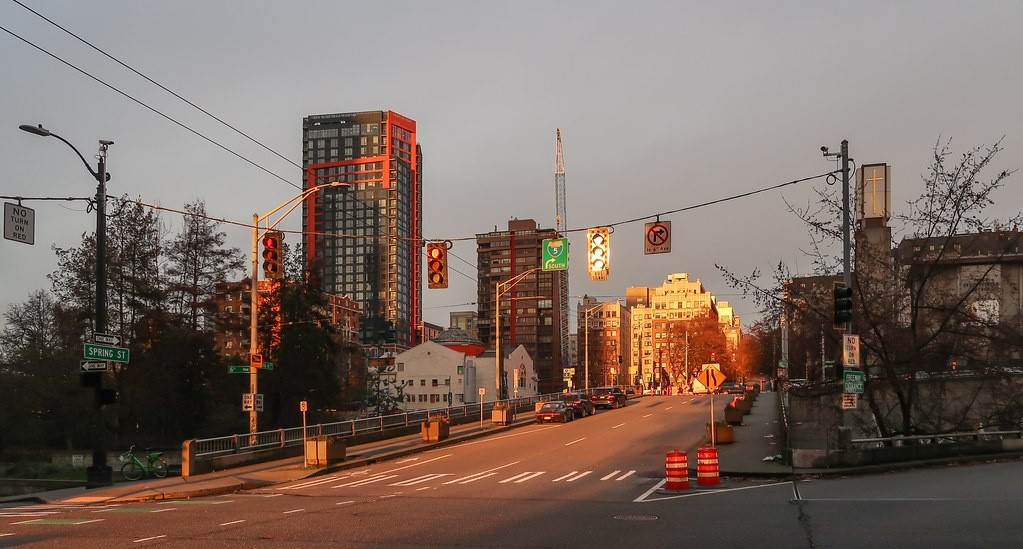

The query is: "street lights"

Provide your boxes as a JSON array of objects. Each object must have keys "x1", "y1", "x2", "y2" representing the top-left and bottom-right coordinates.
[
  {"x1": 584, "y1": 297, "x2": 625, "y2": 396},
  {"x1": 249, "y1": 181, "x2": 350, "y2": 448},
  {"x1": 18, "y1": 122, "x2": 112, "y2": 488}
]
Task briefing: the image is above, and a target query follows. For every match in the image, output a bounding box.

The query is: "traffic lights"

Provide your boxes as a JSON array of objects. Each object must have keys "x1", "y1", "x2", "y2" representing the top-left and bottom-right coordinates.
[
  {"x1": 427, "y1": 243, "x2": 448, "y2": 288},
  {"x1": 833, "y1": 281, "x2": 852, "y2": 328},
  {"x1": 263, "y1": 231, "x2": 282, "y2": 280}
]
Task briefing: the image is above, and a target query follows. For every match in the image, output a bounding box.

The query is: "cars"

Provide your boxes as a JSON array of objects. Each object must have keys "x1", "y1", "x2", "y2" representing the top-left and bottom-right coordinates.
[
  {"x1": 693, "y1": 379, "x2": 810, "y2": 395},
  {"x1": 536, "y1": 402, "x2": 575, "y2": 423}
]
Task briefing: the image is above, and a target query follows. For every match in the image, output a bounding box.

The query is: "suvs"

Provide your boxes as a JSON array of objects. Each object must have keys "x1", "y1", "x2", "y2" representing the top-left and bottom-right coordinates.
[
  {"x1": 592, "y1": 387, "x2": 625, "y2": 408},
  {"x1": 561, "y1": 394, "x2": 595, "y2": 418}
]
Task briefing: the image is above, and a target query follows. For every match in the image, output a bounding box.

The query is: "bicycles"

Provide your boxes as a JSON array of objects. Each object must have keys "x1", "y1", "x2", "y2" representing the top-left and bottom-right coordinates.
[{"x1": 120, "y1": 445, "x2": 170, "y2": 482}]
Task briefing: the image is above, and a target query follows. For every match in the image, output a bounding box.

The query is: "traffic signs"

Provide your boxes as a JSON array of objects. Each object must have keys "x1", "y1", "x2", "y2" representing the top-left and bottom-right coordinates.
[
  {"x1": 588, "y1": 227, "x2": 609, "y2": 275},
  {"x1": 80, "y1": 333, "x2": 130, "y2": 372}
]
